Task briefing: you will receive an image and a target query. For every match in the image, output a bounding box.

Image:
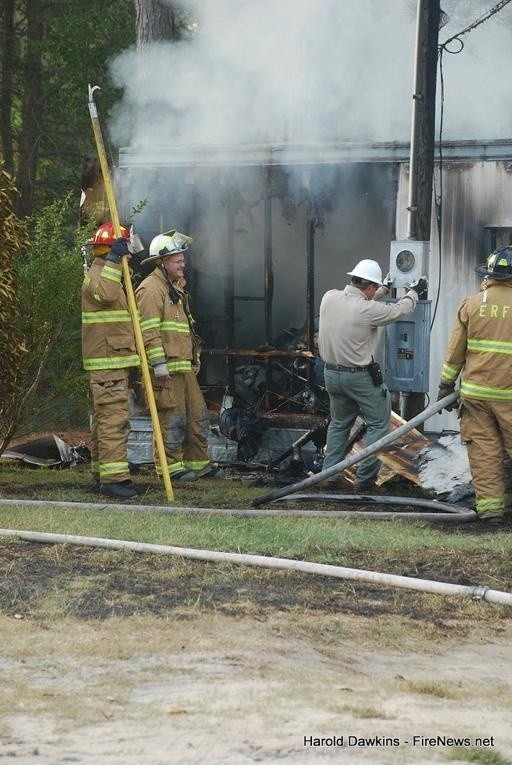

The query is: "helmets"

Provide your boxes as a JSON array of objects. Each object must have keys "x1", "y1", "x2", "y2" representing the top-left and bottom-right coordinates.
[
  {"x1": 84, "y1": 224, "x2": 128, "y2": 246},
  {"x1": 140, "y1": 233, "x2": 188, "y2": 265},
  {"x1": 346, "y1": 259, "x2": 383, "y2": 285},
  {"x1": 475, "y1": 246, "x2": 512, "y2": 280}
]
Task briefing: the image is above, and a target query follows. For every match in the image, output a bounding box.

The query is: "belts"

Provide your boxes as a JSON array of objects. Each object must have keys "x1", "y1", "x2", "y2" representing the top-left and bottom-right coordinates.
[{"x1": 326, "y1": 364, "x2": 367, "y2": 373}]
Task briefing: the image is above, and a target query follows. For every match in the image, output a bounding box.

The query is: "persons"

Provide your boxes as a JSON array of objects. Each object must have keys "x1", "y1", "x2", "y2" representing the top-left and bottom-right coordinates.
[
  {"x1": 317, "y1": 258, "x2": 428, "y2": 498},
  {"x1": 436, "y1": 245, "x2": 512, "y2": 529},
  {"x1": 77, "y1": 222, "x2": 141, "y2": 502},
  {"x1": 133, "y1": 227, "x2": 217, "y2": 483}
]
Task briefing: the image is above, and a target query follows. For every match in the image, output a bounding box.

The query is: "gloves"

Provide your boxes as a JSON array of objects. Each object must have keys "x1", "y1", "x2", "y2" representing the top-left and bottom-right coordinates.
[
  {"x1": 409, "y1": 276, "x2": 428, "y2": 296},
  {"x1": 106, "y1": 237, "x2": 129, "y2": 263},
  {"x1": 382, "y1": 273, "x2": 396, "y2": 291},
  {"x1": 438, "y1": 384, "x2": 459, "y2": 414}
]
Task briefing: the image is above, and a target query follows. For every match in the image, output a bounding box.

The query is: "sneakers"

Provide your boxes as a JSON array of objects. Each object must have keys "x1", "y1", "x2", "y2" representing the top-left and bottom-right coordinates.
[
  {"x1": 354, "y1": 483, "x2": 386, "y2": 495},
  {"x1": 169, "y1": 470, "x2": 196, "y2": 481},
  {"x1": 101, "y1": 484, "x2": 138, "y2": 499},
  {"x1": 202, "y1": 468, "x2": 224, "y2": 480},
  {"x1": 320, "y1": 478, "x2": 353, "y2": 491}
]
